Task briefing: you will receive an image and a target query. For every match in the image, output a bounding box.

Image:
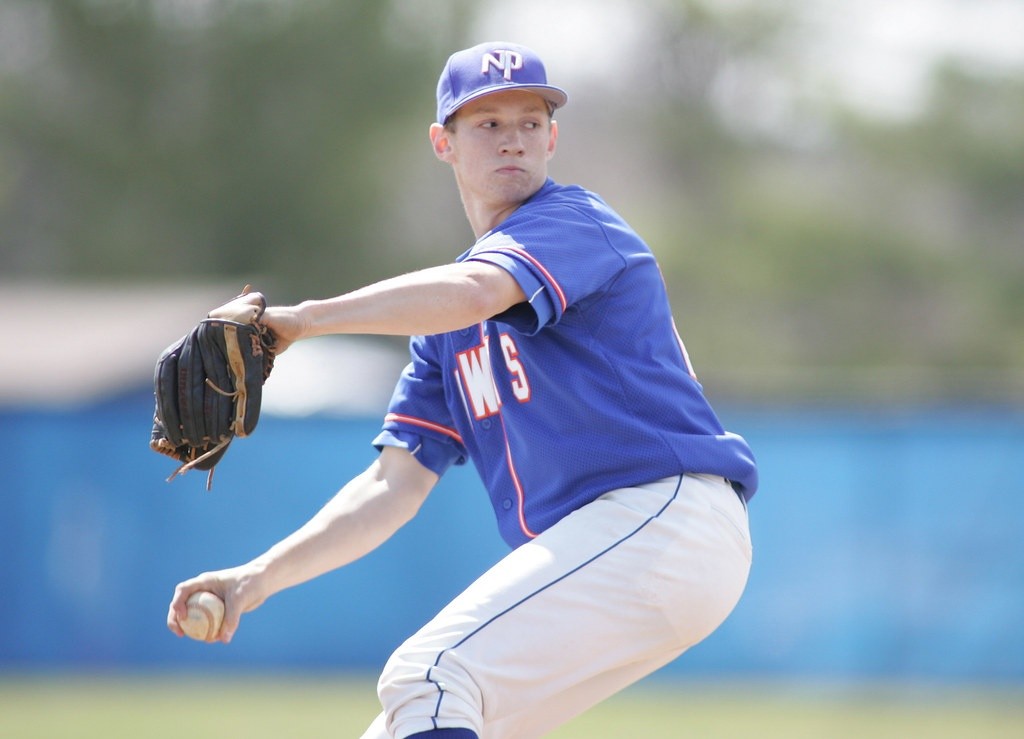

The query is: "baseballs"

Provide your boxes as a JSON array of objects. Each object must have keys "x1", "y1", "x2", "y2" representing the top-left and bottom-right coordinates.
[{"x1": 178, "y1": 588, "x2": 227, "y2": 643}]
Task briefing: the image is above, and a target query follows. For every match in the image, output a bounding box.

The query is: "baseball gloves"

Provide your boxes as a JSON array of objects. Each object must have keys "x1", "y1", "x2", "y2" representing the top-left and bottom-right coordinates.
[{"x1": 153, "y1": 283, "x2": 278, "y2": 474}]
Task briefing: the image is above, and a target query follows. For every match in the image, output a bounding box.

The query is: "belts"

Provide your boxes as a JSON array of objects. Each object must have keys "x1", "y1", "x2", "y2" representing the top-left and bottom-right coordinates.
[{"x1": 725, "y1": 477, "x2": 746, "y2": 509}]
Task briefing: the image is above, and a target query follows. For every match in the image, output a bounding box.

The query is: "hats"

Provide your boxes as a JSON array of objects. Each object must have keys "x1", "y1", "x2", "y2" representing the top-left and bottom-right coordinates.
[{"x1": 436, "y1": 40, "x2": 568, "y2": 128}]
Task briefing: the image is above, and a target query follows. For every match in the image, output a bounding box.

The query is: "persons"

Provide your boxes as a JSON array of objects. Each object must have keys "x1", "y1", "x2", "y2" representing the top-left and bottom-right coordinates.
[{"x1": 148, "y1": 43, "x2": 762, "y2": 739}]
